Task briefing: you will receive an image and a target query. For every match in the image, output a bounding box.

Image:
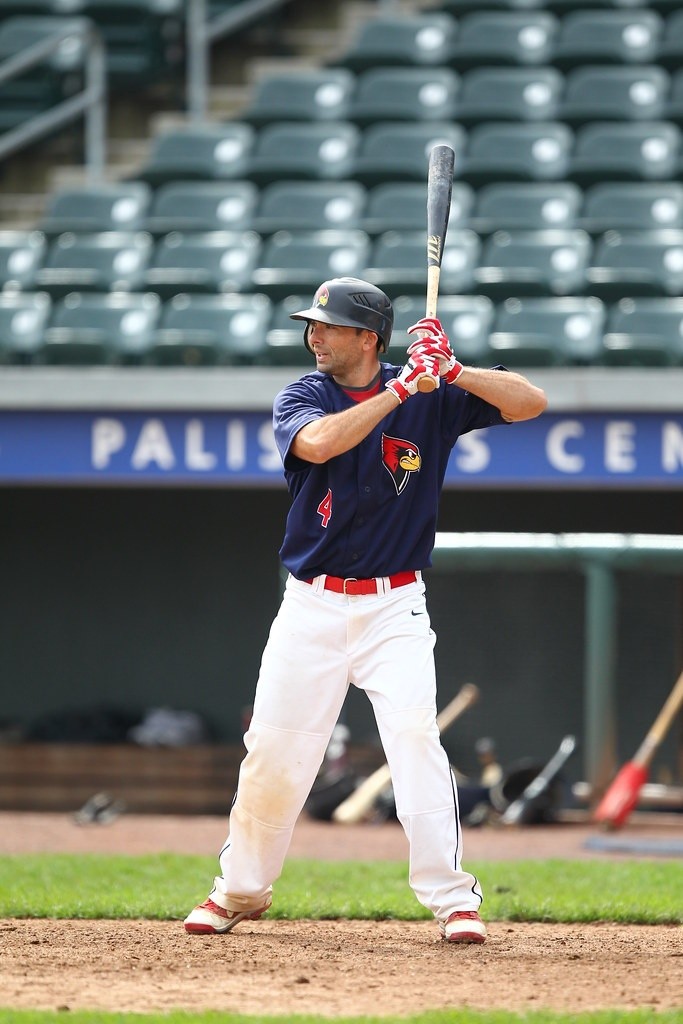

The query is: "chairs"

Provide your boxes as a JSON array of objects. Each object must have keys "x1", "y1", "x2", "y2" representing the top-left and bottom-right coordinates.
[{"x1": 0, "y1": 0, "x2": 683, "y2": 368}]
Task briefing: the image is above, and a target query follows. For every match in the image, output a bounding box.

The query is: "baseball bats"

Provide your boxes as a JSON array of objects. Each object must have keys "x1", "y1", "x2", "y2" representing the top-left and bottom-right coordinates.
[
  {"x1": 594, "y1": 671, "x2": 683, "y2": 830},
  {"x1": 331, "y1": 682, "x2": 481, "y2": 824},
  {"x1": 415, "y1": 145, "x2": 457, "y2": 394},
  {"x1": 504, "y1": 732, "x2": 577, "y2": 829}
]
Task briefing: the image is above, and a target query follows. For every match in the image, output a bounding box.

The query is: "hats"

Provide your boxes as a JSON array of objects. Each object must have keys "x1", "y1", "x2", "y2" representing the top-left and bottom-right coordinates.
[{"x1": 289, "y1": 276, "x2": 394, "y2": 354}]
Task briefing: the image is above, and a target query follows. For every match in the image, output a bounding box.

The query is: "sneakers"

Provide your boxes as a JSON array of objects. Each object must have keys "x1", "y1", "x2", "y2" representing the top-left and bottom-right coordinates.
[
  {"x1": 439, "y1": 911, "x2": 487, "y2": 944},
  {"x1": 183, "y1": 897, "x2": 272, "y2": 934}
]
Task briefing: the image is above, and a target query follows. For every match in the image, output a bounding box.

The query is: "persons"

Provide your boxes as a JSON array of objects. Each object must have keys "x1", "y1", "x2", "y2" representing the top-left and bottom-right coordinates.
[{"x1": 184, "y1": 276, "x2": 547, "y2": 944}]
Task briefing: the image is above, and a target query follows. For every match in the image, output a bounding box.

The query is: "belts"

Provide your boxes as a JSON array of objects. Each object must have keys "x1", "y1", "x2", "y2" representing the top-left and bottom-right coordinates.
[{"x1": 304, "y1": 570, "x2": 417, "y2": 597}]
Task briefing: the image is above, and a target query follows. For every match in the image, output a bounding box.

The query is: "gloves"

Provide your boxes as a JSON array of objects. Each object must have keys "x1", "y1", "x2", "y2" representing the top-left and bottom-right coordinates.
[
  {"x1": 406, "y1": 318, "x2": 464, "y2": 385},
  {"x1": 384, "y1": 353, "x2": 440, "y2": 404}
]
